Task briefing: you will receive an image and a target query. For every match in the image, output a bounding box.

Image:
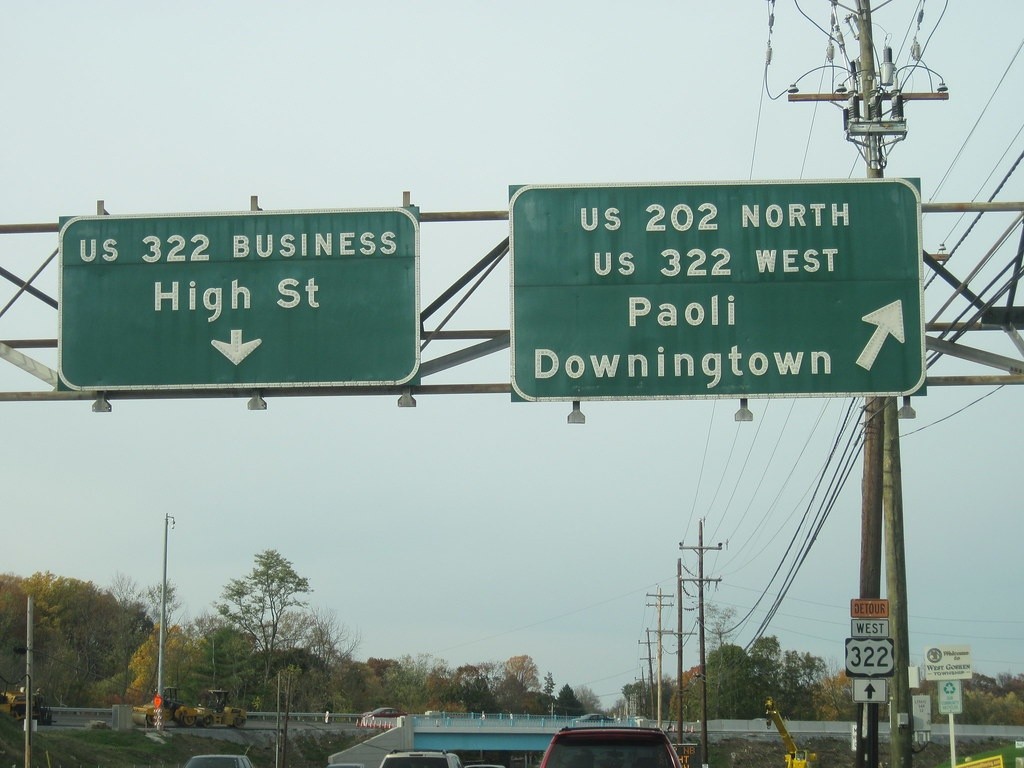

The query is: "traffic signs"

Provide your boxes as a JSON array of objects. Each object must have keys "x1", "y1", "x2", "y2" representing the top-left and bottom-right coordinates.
[
  {"x1": 58, "y1": 203, "x2": 424, "y2": 392},
  {"x1": 504, "y1": 178, "x2": 931, "y2": 402},
  {"x1": 843, "y1": 596, "x2": 896, "y2": 703}
]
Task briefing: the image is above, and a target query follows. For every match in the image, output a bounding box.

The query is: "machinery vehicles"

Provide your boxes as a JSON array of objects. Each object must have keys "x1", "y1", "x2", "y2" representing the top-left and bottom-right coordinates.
[
  {"x1": 181, "y1": 687, "x2": 248, "y2": 729},
  {"x1": 1, "y1": 684, "x2": 56, "y2": 728},
  {"x1": 130, "y1": 681, "x2": 185, "y2": 727},
  {"x1": 763, "y1": 696, "x2": 818, "y2": 768}
]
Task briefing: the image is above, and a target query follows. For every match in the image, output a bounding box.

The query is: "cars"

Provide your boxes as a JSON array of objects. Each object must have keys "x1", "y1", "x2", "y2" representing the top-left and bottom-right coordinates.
[
  {"x1": 180, "y1": 747, "x2": 511, "y2": 767},
  {"x1": 362, "y1": 706, "x2": 409, "y2": 718},
  {"x1": 535, "y1": 723, "x2": 684, "y2": 768}
]
